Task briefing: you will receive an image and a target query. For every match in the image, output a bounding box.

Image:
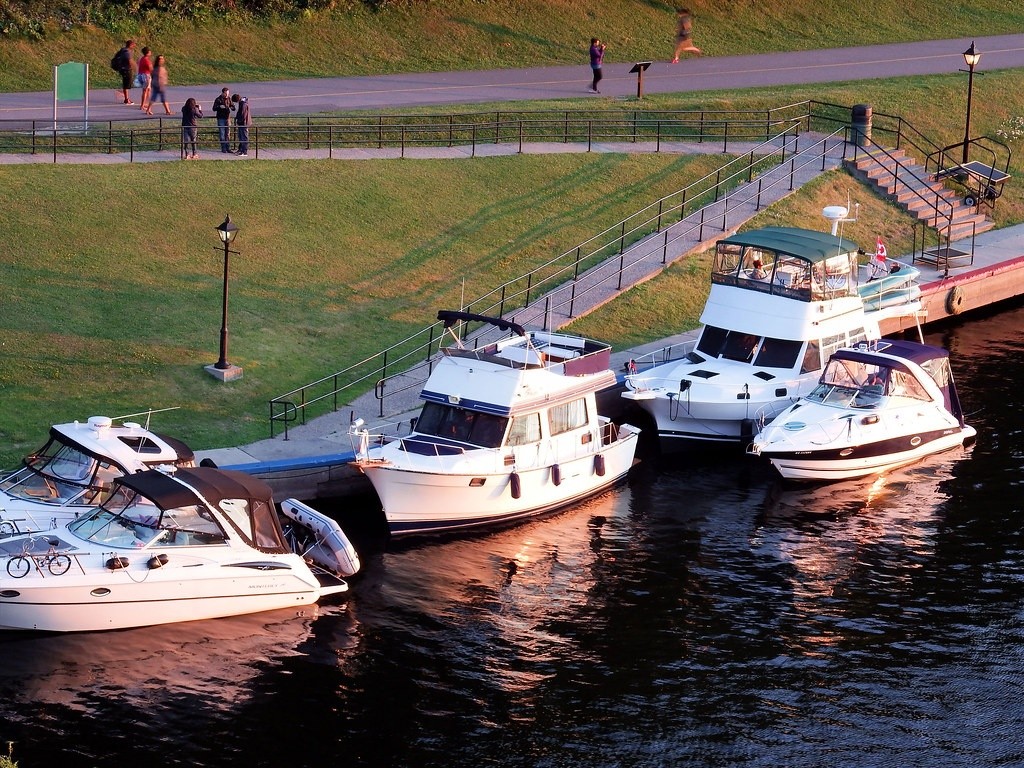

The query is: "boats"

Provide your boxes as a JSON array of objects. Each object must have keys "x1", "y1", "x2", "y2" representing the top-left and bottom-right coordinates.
[
  {"x1": 0, "y1": 465, "x2": 350, "y2": 634},
  {"x1": 863, "y1": 285, "x2": 922, "y2": 311},
  {"x1": 348, "y1": 307, "x2": 639, "y2": 540},
  {"x1": 0, "y1": 415, "x2": 195, "y2": 537},
  {"x1": 859, "y1": 267, "x2": 919, "y2": 298},
  {"x1": 281, "y1": 499, "x2": 361, "y2": 577},
  {"x1": 752, "y1": 337, "x2": 978, "y2": 482},
  {"x1": 619, "y1": 225, "x2": 879, "y2": 442}
]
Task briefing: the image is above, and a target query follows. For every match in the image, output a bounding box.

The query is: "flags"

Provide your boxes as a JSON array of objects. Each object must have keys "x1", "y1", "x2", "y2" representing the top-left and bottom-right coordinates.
[{"x1": 877, "y1": 239, "x2": 886, "y2": 264}]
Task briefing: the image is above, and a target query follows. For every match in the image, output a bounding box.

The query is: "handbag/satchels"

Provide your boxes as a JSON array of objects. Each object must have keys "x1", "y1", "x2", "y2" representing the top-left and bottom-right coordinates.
[{"x1": 131, "y1": 74, "x2": 147, "y2": 89}]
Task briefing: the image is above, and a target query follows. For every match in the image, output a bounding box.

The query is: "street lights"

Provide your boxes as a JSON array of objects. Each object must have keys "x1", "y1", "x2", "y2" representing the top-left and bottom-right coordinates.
[
  {"x1": 962, "y1": 40, "x2": 983, "y2": 163},
  {"x1": 215, "y1": 214, "x2": 238, "y2": 370}
]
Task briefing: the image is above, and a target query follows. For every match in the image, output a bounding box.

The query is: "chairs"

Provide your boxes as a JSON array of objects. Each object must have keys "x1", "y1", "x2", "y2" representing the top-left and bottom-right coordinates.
[{"x1": 165, "y1": 532, "x2": 189, "y2": 546}]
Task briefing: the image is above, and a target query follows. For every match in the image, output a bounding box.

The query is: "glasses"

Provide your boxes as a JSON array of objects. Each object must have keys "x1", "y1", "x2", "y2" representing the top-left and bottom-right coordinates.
[
  {"x1": 760, "y1": 264, "x2": 763, "y2": 267},
  {"x1": 602, "y1": 43, "x2": 606, "y2": 46}
]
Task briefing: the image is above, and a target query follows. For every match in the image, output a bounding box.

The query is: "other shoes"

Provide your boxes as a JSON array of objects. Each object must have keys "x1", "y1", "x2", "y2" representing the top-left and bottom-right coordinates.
[
  {"x1": 587, "y1": 82, "x2": 598, "y2": 94},
  {"x1": 124, "y1": 98, "x2": 135, "y2": 105},
  {"x1": 146, "y1": 110, "x2": 153, "y2": 116},
  {"x1": 185, "y1": 154, "x2": 199, "y2": 160},
  {"x1": 234, "y1": 149, "x2": 248, "y2": 157},
  {"x1": 221, "y1": 150, "x2": 234, "y2": 154},
  {"x1": 166, "y1": 110, "x2": 175, "y2": 115},
  {"x1": 671, "y1": 58, "x2": 679, "y2": 64},
  {"x1": 140, "y1": 104, "x2": 149, "y2": 110}
]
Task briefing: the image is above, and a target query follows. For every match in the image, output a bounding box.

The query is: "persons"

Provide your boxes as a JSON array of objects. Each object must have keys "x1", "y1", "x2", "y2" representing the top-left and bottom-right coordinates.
[
  {"x1": 673, "y1": 9, "x2": 701, "y2": 65},
  {"x1": 146, "y1": 55, "x2": 176, "y2": 115},
  {"x1": 213, "y1": 87, "x2": 236, "y2": 154},
  {"x1": 117, "y1": 40, "x2": 137, "y2": 104},
  {"x1": 182, "y1": 98, "x2": 203, "y2": 160},
  {"x1": 136, "y1": 46, "x2": 152, "y2": 111},
  {"x1": 588, "y1": 37, "x2": 607, "y2": 95},
  {"x1": 232, "y1": 93, "x2": 249, "y2": 156},
  {"x1": 751, "y1": 261, "x2": 765, "y2": 281}
]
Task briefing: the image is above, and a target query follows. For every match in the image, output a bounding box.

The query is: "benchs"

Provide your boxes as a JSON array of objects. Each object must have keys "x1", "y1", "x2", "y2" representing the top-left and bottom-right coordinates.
[
  {"x1": 55, "y1": 469, "x2": 104, "y2": 505},
  {"x1": 492, "y1": 333, "x2": 586, "y2": 370}
]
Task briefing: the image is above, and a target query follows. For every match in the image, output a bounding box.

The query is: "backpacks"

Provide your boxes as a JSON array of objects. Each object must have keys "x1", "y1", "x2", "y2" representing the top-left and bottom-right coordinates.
[{"x1": 110, "y1": 49, "x2": 126, "y2": 75}]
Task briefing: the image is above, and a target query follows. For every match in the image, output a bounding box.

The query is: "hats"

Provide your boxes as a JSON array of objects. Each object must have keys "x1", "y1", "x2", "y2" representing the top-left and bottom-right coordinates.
[{"x1": 753, "y1": 260, "x2": 761, "y2": 269}]
